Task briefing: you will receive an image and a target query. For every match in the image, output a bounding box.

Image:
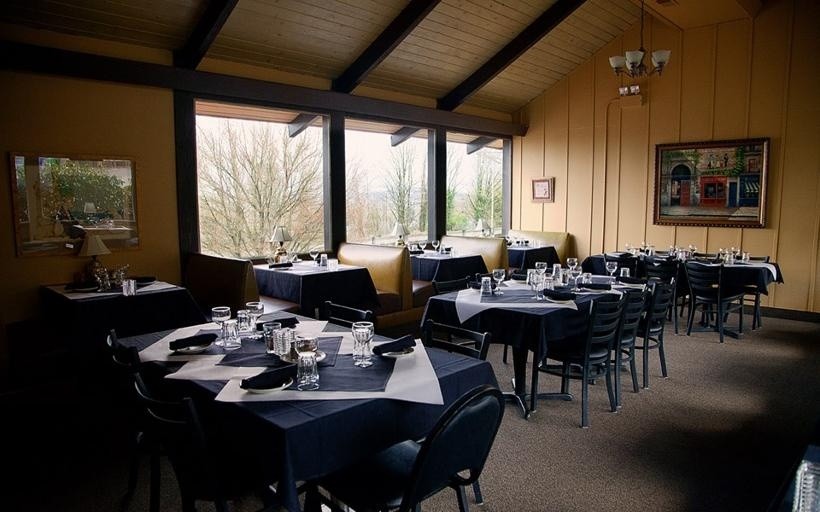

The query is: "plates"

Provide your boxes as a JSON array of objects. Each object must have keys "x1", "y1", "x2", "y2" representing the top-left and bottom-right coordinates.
[
  {"x1": 281, "y1": 350, "x2": 327, "y2": 363},
  {"x1": 176, "y1": 342, "x2": 214, "y2": 352},
  {"x1": 659, "y1": 254, "x2": 669, "y2": 258},
  {"x1": 609, "y1": 252, "x2": 626, "y2": 255},
  {"x1": 128, "y1": 275, "x2": 157, "y2": 287},
  {"x1": 545, "y1": 296, "x2": 574, "y2": 303},
  {"x1": 239, "y1": 378, "x2": 293, "y2": 394},
  {"x1": 382, "y1": 347, "x2": 414, "y2": 358},
  {"x1": 591, "y1": 275, "x2": 616, "y2": 284}
]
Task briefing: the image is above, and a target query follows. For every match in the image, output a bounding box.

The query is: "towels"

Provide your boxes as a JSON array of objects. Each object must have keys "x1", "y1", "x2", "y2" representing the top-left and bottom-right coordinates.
[
  {"x1": 544, "y1": 276, "x2": 648, "y2": 301},
  {"x1": 169, "y1": 317, "x2": 415, "y2": 389},
  {"x1": 473, "y1": 274, "x2": 526, "y2": 289}
]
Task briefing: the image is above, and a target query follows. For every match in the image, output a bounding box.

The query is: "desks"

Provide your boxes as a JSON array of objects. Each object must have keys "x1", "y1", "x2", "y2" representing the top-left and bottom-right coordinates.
[{"x1": 38, "y1": 276, "x2": 188, "y2": 305}]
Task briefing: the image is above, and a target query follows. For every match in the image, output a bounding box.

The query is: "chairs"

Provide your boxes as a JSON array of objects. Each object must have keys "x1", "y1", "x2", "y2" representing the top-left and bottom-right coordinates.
[
  {"x1": 529, "y1": 294, "x2": 617, "y2": 429},
  {"x1": 320, "y1": 300, "x2": 492, "y2": 360},
  {"x1": 588, "y1": 277, "x2": 676, "y2": 391},
  {"x1": 562, "y1": 290, "x2": 639, "y2": 406},
  {"x1": 305, "y1": 384, "x2": 505, "y2": 512},
  {"x1": 104, "y1": 328, "x2": 277, "y2": 512},
  {"x1": 605, "y1": 246, "x2": 771, "y2": 343}
]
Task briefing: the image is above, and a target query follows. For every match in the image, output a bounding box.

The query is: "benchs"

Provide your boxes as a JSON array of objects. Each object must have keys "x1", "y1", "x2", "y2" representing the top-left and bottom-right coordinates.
[
  {"x1": 439, "y1": 235, "x2": 508, "y2": 274},
  {"x1": 188, "y1": 253, "x2": 301, "y2": 313},
  {"x1": 338, "y1": 243, "x2": 433, "y2": 328},
  {"x1": 508, "y1": 229, "x2": 570, "y2": 268}
]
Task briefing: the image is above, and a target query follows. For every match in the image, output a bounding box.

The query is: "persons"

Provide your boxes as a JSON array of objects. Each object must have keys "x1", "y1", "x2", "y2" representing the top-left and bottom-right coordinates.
[{"x1": 707, "y1": 153, "x2": 728, "y2": 169}]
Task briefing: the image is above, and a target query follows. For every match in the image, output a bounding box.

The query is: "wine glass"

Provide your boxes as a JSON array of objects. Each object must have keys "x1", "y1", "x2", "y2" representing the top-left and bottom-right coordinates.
[
  {"x1": 246, "y1": 302, "x2": 264, "y2": 339},
  {"x1": 567, "y1": 258, "x2": 578, "y2": 279},
  {"x1": 689, "y1": 241, "x2": 697, "y2": 255},
  {"x1": 493, "y1": 269, "x2": 506, "y2": 295},
  {"x1": 606, "y1": 262, "x2": 618, "y2": 275},
  {"x1": 732, "y1": 246, "x2": 739, "y2": 259},
  {"x1": 641, "y1": 241, "x2": 649, "y2": 253},
  {"x1": 295, "y1": 336, "x2": 319, "y2": 390},
  {"x1": 212, "y1": 307, "x2": 232, "y2": 346},
  {"x1": 570, "y1": 266, "x2": 582, "y2": 291},
  {"x1": 419, "y1": 242, "x2": 426, "y2": 251},
  {"x1": 648, "y1": 241, "x2": 655, "y2": 256},
  {"x1": 535, "y1": 262, "x2": 548, "y2": 282},
  {"x1": 352, "y1": 321, "x2": 374, "y2": 368},
  {"x1": 432, "y1": 240, "x2": 439, "y2": 255},
  {"x1": 625, "y1": 240, "x2": 633, "y2": 251},
  {"x1": 310, "y1": 251, "x2": 319, "y2": 267},
  {"x1": 720, "y1": 248, "x2": 728, "y2": 258}
]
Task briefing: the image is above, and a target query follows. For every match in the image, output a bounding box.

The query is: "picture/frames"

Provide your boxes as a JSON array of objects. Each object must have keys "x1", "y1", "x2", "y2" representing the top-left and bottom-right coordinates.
[
  {"x1": 652, "y1": 138, "x2": 769, "y2": 229},
  {"x1": 10, "y1": 150, "x2": 141, "y2": 260}
]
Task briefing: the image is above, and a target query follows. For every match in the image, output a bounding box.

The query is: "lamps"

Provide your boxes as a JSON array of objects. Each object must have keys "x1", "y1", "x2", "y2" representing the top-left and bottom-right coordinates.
[
  {"x1": 609, "y1": 0, "x2": 672, "y2": 79},
  {"x1": 76, "y1": 234, "x2": 112, "y2": 284}
]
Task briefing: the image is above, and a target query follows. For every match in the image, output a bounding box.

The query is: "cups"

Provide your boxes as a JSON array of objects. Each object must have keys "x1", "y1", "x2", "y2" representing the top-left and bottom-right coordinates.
[
  {"x1": 742, "y1": 252, "x2": 751, "y2": 262},
  {"x1": 481, "y1": 277, "x2": 492, "y2": 297},
  {"x1": 620, "y1": 268, "x2": 630, "y2": 277},
  {"x1": 537, "y1": 282, "x2": 544, "y2": 300},
  {"x1": 412, "y1": 245, "x2": 417, "y2": 250},
  {"x1": 553, "y1": 264, "x2": 561, "y2": 279},
  {"x1": 328, "y1": 259, "x2": 338, "y2": 271},
  {"x1": 530, "y1": 240, "x2": 534, "y2": 247},
  {"x1": 678, "y1": 248, "x2": 685, "y2": 263},
  {"x1": 262, "y1": 322, "x2": 281, "y2": 353},
  {"x1": 516, "y1": 241, "x2": 520, "y2": 247},
  {"x1": 297, "y1": 355, "x2": 319, "y2": 391},
  {"x1": 535, "y1": 240, "x2": 540, "y2": 248},
  {"x1": 223, "y1": 319, "x2": 241, "y2": 350},
  {"x1": 524, "y1": 240, "x2": 529, "y2": 246},
  {"x1": 353, "y1": 330, "x2": 371, "y2": 361},
  {"x1": 321, "y1": 254, "x2": 327, "y2": 267},
  {"x1": 583, "y1": 273, "x2": 592, "y2": 284},
  {"x1": 122, "y1": 280, "x2": 136, "y2": 296},
  {"x1": 545, "y1": 279, "x2": 554, "y2": 300},
  {"x1": 444, "y1": 247, "x2": 451, "y2": 254},
  {"x1": 526, "y1": 269, "x2": 536, "y2": 288},
  {"x1": 237, "y1": 310, "x2": 257, "y2": 339}
]
